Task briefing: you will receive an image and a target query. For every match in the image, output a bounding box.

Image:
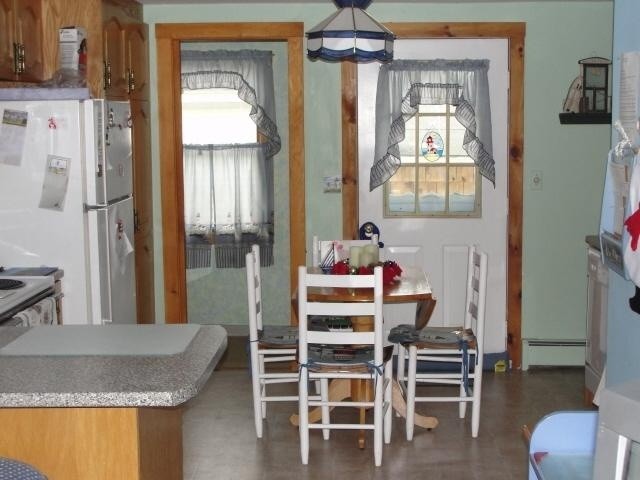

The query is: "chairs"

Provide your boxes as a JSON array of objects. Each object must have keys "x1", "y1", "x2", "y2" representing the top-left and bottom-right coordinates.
[
  {"x1": 298, "y1": 267, "x2": 393, "y2": 467},
  {"x1": 395, "y1": 244, "x2": 487, "y2": 442},
  {"x1": 246, "y1": 233, "x2": 330, "y2": 439},
  {"x1": 314, "y1": 237, "x2": 381, "y2": 267}
]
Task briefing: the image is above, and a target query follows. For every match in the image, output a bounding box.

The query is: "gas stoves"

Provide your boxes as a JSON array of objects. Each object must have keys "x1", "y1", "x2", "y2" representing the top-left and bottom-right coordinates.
[{"x1": 0, "y1": 276, "x2": 56, "y2": 324}]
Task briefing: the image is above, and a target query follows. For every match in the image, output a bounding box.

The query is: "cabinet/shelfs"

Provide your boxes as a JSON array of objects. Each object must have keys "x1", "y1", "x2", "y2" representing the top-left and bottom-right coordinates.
[
  {"x1": 0, "y1": 0, "x2": 43, "y2": 87},
  {"x1": 131, "y1": 0, "x2": 155, "y2": 324},
  {"x1": 44, "y1": 0, "x2": 131, "y2": 101},
  {"x1": 584, "y1": 248, "x2": 608, "y2": 377}
]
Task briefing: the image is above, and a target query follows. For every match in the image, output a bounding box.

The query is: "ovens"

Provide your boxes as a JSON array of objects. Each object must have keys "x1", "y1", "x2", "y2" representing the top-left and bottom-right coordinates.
[{"x1": 0, "y1": 279, "x2": 66, "y2": 324}]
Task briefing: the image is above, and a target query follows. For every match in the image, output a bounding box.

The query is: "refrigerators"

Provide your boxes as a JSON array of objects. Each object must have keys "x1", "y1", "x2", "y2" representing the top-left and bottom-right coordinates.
[{"x1": 0, "y1": 98, "x2": 138, "y2": 324}]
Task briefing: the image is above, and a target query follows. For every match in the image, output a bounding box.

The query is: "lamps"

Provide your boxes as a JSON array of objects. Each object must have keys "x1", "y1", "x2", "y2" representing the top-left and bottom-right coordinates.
[{"x1": 306, "y1": 1, "x2": 394, "y2": 63}]
